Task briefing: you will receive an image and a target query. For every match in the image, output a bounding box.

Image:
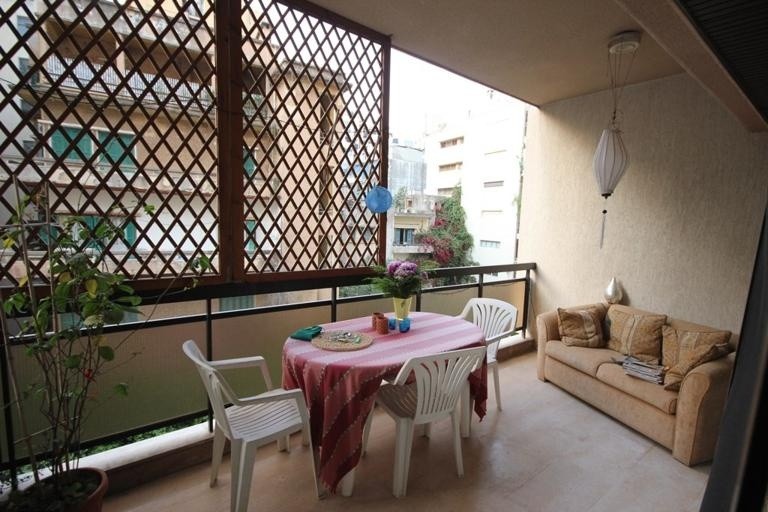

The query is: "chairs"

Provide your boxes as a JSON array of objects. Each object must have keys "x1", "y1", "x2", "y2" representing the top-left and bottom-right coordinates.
[
  {"x1": 182, "y1": 339, "x2": 327, "y2": 512},
  {"x1": 360, "y1": 345, "x2": 486, "y2": 498},
  {"x1": 451, "y1": 298, "x2": 518, "y2": 433}
]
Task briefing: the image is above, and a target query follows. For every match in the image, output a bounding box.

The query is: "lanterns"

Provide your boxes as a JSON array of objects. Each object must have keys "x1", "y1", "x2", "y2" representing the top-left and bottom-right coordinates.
[{"x1": 592, "y1": 129, "x2": 629, "y2": 250}]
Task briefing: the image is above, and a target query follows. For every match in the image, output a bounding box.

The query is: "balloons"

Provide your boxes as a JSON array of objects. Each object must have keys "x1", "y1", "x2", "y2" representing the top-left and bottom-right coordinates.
[{"x1": 365, "y1": 186, "x2": 392, "y2": 214}]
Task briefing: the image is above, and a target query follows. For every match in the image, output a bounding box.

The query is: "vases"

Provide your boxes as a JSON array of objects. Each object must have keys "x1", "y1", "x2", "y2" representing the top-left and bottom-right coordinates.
[{"x1": 393, "y1": 296, "x2": 412, "y2": 320}]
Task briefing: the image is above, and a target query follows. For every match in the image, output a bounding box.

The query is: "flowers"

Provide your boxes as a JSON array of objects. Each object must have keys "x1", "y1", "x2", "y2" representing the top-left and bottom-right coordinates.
[{"x1": 362, "y1": 261, "x2": 428, "y2": 298}]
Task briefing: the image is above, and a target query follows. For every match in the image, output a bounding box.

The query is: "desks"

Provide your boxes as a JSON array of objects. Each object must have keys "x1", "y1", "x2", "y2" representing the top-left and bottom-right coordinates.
[{"x1": 282, "y1": 312, "x2": 487, "y2": 497}]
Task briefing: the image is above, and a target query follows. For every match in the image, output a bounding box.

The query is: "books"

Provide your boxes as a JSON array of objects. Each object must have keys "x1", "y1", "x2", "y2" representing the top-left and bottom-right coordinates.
[{"x1": 623, "y1": 355, "x2": 665, "y2": 384}]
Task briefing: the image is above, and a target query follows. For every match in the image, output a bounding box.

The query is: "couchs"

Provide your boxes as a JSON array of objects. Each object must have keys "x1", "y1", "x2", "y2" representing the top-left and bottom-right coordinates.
[{"x1": 535, "y1": 303, "x2": 740, "y2": 467}]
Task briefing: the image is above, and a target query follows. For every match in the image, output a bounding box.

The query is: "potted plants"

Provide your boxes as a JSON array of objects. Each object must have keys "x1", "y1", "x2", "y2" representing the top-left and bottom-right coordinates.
[{"x1": 0, "y1": 195, "x2": 211, "y2": 512}]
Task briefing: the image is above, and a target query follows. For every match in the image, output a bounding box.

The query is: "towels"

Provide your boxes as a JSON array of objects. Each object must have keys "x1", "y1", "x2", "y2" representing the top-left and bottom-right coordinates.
[{"x1": 290, "y1": 325, "x2": 322, "y2": 341}]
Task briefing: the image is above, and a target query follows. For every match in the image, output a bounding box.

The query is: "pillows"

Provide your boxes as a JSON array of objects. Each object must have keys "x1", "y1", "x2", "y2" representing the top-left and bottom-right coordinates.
[
  {"x1": 663, "y1": 343, "x2": 729, "y2": 391},
  {"x1": 661, "y1": 324, "x2": 731, "y2": 366},
  {"x1": 557, "y1": 307, "x2": 607, "y2": 348},
  {"x1": 607, "y1": 309, "x2": 667, "y2": 365}
]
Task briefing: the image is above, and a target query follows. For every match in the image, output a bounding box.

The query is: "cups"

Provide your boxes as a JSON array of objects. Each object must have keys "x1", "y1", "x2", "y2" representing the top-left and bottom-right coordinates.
[
  {"x1": 389, "y1": 318, "x2": 396, "y2": 329},
  {"x1": 372, "y1": 312, "x2": 384, "y2": 328},
  {"x1": 403, "y1": 318, "x2": 410, "y2": 330},
  {"x1": 399, "y1": 322, "x2": 407, "y2": 332},
  {"x1": 376, "y1": 316, "x2": 387, "y2": 334}
]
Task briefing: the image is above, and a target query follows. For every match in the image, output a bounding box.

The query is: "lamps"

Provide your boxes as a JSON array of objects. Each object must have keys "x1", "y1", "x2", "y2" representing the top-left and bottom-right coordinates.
[{"x1": 591, "y1": 30, "x2": 649, "y2": 248}]
